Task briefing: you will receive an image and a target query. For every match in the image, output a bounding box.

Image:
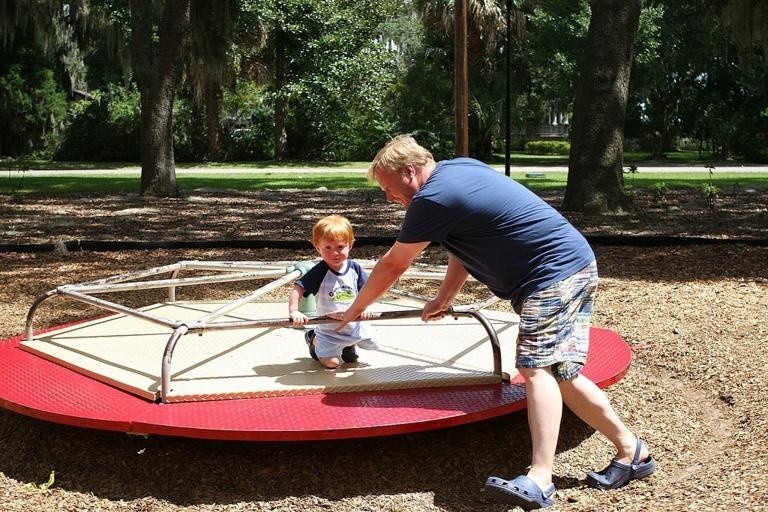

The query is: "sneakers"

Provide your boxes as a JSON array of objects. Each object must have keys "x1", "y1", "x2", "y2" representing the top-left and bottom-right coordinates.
[{"x1": 341, "y1": 345, "x2": 358, "y2": 362}]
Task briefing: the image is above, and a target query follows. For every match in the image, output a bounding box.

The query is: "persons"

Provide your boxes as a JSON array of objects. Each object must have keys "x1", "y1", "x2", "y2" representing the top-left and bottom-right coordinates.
[
  {"x1": 320, "y1": 135, "x2": 658, "y2": 507},
  {"x1": 288, "y1": 213, "x2": 371, "y2": 370}
]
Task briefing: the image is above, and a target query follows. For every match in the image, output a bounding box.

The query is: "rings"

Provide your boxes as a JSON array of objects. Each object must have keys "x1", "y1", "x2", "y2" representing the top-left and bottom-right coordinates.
[{"x1": 340, "y1": 313, "x2": 344, "y2": 320}]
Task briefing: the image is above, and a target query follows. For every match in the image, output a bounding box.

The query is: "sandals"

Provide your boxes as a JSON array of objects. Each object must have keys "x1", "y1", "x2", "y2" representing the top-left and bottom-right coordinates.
[
  {"x1": 304, "y1": 329, "x2": 319, "y2": 361},
  {"x1": 586, "y1": 439, "x2": 655, "y2": 491},
  {"x1": 485, "y1": 474, "x2": 557, "y2": 508}
]
